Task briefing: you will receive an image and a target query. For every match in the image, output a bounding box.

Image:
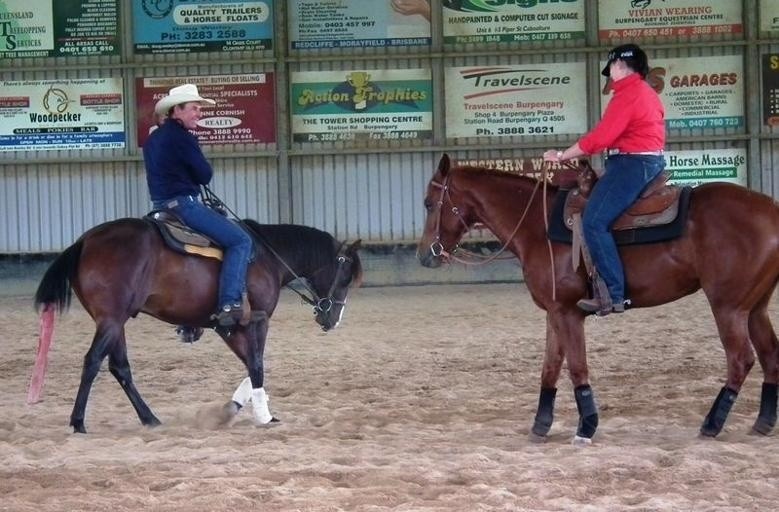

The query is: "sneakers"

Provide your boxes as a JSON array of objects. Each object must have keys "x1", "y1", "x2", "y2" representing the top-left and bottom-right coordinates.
[
  {"x1": 218, "y1": 303, "x2": 267, "y2": 327},
  {"x1": 178, "y1": 326, "x2": 204, "y2": 343},
  {"x1": 576, "y1": 297, "x2": 625, "y2": 313}
]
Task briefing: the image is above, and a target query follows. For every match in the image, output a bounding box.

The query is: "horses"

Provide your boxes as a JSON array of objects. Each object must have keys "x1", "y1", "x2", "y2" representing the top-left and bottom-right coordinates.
[
  {"x1": 28, "y1": 216, "x2": 364, "y2": 433},
  {"x1": 414, "y1": 152, "x2": 779, "y2": 445}
]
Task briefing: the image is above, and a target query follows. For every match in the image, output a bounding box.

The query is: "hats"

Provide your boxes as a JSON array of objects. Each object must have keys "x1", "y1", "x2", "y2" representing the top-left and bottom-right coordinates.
[
  {"x1": 154, "y1": 84, "x2": 216, "y2": 119},
  {"x1": 602, "y1": 44, "x2": 645, "y2": 77}
]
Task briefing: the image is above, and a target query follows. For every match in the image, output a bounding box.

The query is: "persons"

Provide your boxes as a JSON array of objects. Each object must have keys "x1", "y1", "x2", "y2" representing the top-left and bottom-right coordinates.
[
  {"x1": 543, "y1": 43, "x2": 665, "y2": 314},
  {"x1": 142, "y1": 83, "x2": 268, "y2": 341}
]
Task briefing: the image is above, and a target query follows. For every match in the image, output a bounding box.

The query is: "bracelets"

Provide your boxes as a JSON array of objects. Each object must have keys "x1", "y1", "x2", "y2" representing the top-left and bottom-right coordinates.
[{"x1": 555, "y1": 150, "x2": 564, "y2": 160}]
